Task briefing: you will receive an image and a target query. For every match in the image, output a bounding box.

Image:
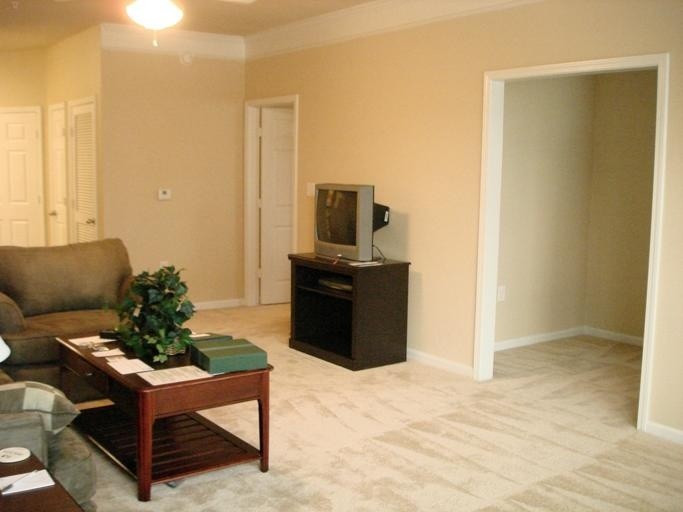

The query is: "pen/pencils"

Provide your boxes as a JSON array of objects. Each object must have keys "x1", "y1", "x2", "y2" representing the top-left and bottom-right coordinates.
[{"x1": 0, "y1": 469, "x2": 37, "y2": 494}]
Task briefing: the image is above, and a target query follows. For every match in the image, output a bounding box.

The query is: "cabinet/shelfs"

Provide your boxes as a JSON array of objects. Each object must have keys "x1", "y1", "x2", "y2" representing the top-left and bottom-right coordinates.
[{"x1": 287, "y1": 252, "x2": 411, "y2": 371}]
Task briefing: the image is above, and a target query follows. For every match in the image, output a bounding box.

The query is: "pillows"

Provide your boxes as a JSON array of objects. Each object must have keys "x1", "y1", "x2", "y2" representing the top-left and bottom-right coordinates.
[{"x1": 0, "y1": 381, "x2": 81, "y2": 441}]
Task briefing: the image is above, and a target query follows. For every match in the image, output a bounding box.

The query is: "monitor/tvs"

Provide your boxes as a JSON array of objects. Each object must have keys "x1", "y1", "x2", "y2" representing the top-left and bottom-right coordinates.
[{"x1": 313, "y1": 183, "x2": 390, "y2": 262}]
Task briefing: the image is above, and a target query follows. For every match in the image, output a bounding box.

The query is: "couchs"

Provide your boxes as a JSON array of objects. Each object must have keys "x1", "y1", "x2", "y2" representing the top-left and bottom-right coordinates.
[{"x1": 0, "y1": 339, "x2": 97, "y2": 512}]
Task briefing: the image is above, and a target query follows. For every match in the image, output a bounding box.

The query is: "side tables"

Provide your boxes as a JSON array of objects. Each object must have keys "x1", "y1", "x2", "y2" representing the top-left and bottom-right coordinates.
[{"x1": 0, "y1": 449, "x2": 85, "y2": 512}]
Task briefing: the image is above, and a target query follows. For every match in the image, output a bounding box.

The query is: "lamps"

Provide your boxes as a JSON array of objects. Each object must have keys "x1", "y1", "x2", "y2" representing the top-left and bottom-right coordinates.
[{"x1": 125, "y1": 0, "x2": 183, "y2": 48}]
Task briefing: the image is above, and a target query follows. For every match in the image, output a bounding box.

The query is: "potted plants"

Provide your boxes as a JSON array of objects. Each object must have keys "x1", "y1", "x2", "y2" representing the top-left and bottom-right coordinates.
[{"x1": 108, "y1": 265, "x2": 197, "y2": 362}]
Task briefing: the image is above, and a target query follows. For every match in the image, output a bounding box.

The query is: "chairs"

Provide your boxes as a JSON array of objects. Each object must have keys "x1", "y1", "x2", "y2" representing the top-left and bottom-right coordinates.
[{"x1": 0, "y1": 238, "x2": 145, "y2": 404}]
